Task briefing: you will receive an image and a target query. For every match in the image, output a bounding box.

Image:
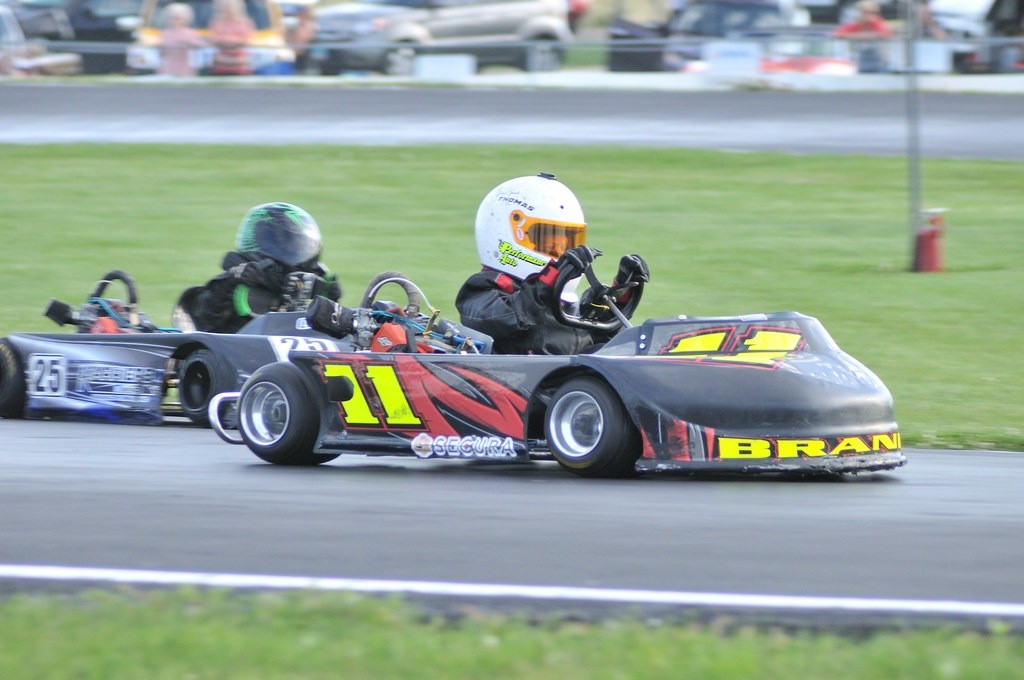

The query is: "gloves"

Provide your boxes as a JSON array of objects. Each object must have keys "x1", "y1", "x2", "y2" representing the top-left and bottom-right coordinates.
[
  {"x1": 231, "y1": 259, "x2": 274, "y2": 290},
  {"x1": 555, "y1": 243, "x2": 603, "y2": 270},
  {"x1": 615, "y1": 253, "x2": 650, "y2": 288},
  {"x1": 282, "y1": 270, "x2": 330, "y2": 303}
]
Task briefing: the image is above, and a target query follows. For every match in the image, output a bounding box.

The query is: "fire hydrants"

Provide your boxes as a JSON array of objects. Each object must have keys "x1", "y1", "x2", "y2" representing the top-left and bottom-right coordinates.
[{"x1": 912, "y1": 218, "x2": 944, "y2": 273}]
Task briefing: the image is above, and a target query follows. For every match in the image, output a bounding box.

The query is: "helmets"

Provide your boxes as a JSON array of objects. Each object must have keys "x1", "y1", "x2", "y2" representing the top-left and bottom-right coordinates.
[
  {"x1": 473, "y1": 171, "x2": 588, "y2": 281},
  {"x1": 233, "y1": 200, "x2": 323, "y2": 271}
]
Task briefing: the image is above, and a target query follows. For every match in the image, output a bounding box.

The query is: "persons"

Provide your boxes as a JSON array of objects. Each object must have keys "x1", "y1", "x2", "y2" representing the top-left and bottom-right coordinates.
[
  {"x1": 455, "y1": 173, "x2": 649, "y2": 356},
  {"x1": 833, "y1": 0, "x2": 894, "y2": 73},
  {"x1": 207, "y1": 0, "x2": 255, "y2": 77},
  {"x1": 142, "y1": 4, "x2": 206, "y2": 76},
  {"x1": 189, "y1": 202, "x2": 341, "y2": 335},
  {"x1": 985, "y1": 1, "x2": 1024, "y2": 73},
  {"x1": 0, "y1": 43, "x2": 79, "y2": 80},
  {"x1": 911, "y1": 0, "x2": 948, "y2": 39},
  {"x1": 284, "y1": 9, "x2": 319, "y2": 74}
]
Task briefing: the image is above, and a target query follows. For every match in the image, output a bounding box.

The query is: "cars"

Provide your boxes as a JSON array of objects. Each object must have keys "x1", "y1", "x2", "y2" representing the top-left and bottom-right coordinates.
[
  {"x1": 606, "y1": 0, "x2": 1023, "y2": 71},
  {"x1": 0, "y1": 1, "x2": 312, "y2": 75}
]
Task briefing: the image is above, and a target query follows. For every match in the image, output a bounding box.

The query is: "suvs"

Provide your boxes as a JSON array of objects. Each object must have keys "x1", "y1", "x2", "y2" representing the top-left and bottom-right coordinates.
[{"x1": 312, "y1": 1, "x2": 589, "y2": 76}]
